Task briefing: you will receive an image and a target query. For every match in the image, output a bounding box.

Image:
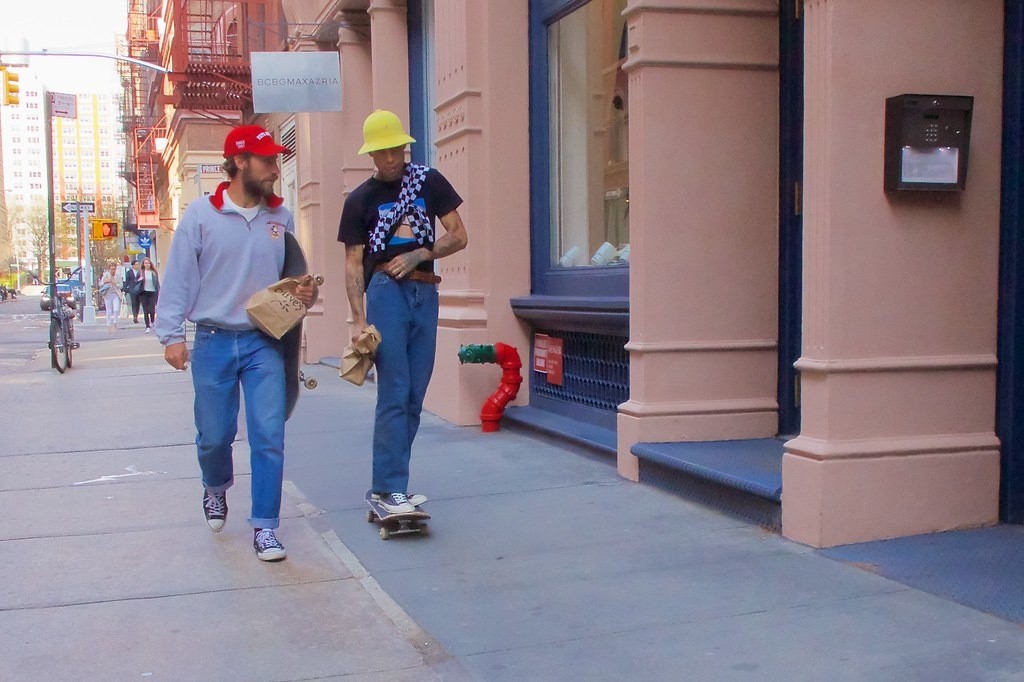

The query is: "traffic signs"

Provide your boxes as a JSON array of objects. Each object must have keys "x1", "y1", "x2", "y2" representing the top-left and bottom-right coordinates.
[
  {"x1": 61, "y1": 201, "x2": 94, "y2": 214},
  {"x1": 46, "y1": 91, "x2": 79, "y2": 118}
]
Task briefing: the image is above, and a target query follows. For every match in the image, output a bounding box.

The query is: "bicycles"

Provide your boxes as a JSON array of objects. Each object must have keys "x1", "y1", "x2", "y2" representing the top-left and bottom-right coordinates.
[{"x1": 26, "y1": 266, "x2": 82, "y2": 374}]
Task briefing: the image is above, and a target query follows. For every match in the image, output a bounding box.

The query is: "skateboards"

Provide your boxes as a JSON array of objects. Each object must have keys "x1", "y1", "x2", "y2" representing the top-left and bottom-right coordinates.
[
  {"x1": 364, "y1": 488, "x2": 431, "y2": 541},
  {"x1": 281, "y1": 230, "x2": 325, "y2": 423}
]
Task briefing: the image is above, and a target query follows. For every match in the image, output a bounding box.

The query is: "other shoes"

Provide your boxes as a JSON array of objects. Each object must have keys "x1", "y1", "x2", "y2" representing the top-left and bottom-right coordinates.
[
  {"x1": 150, "y1": 321, "x2": 154, "y2": 328},
  {"x1": 145, "y1": 327, "x2": 151, "y2": 333},
  {"x1": 113, "y1": 326, "x2": 118, "y2": 333},
  {"x1": 106, "y1": 328, "x2": 112, "y2": 334},
  {"x1": 133, "y1": 318, "x2": 139, "y2": 324}
]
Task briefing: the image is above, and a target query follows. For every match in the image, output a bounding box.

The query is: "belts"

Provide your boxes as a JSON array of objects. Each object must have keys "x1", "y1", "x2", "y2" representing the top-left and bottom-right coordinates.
[{"x1": 373, "y1": 262, "x2": 442, "y2": 284}]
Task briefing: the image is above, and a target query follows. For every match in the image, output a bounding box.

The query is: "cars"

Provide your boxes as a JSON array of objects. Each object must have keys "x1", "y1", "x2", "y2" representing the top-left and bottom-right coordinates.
[
  {"x1": 55, "y1": 279, "x2": 81, "y2": 300},
  {"x1": 40, "y1": 284, "x2": 77, "y2": 311}
]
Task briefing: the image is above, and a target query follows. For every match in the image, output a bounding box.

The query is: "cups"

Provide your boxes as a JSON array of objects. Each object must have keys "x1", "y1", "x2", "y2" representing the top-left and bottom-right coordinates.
[
  {"x1": 591, "y1": 241, "x2": 617, "y2": 265},
  {"x1": 560, "y1": 246, "x2": 583, "y2": 267},
  {"x1": 619, "y1": 244, "x2": 630, "y2": 264}
]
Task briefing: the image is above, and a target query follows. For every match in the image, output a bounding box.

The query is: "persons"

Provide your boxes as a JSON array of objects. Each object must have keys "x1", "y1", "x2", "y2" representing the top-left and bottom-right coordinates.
[
  {"x1": 154, "y1": 123, "x2": 319, "y2": 560},
  {"x1": 99, "y1": 263, "x2": 124, "y2": 326},
  {"x1": 337, "y1": 108, "x2": 468, "y2": 514},
  {"x1": 123, "y1": 260, "x2": 140, "y2": 323},
  {"x1": 135, "y1": 257, "x2": 160, "y2": 333}
]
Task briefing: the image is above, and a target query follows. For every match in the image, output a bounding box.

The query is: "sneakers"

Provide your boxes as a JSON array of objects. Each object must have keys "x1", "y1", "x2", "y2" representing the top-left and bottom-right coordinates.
[
  {"x1": 377, "y1": 492, "x2": 415, "y2": 514},
  {"x1": 254, "y1": 528, "x2": 286, "y2": 561},
  {"x1": 202, "y1": 487, "x2": 228, "y2": 532},
  {"x1": 371, "y1": 493, "x2": 428, "y2": 506}
]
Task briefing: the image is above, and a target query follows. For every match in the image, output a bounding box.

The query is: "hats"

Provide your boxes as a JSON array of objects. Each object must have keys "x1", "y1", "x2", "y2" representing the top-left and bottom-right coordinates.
[
  {"x1": 358, "y1": 109, "x2": 417, "y2": 154},
  {"x1": 223, "y1": 124, "x2": 292, "y2": 158}
]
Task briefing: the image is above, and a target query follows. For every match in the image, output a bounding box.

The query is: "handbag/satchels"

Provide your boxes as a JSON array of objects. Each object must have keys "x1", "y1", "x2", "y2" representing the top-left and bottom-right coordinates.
[
  {"x1": 134, "y1": 279, "x2": 144, "y2": 294},
  {"x1": 99, "y1": 284, "x2": 109, "y2": 296},
  {"x1": 120, "y1": 298, "x2": 129, "y2": 319}
]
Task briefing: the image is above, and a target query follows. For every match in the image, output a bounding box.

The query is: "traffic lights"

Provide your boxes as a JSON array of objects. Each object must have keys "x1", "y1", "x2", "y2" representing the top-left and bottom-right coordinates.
[
  {"x1": 101, "y1": 221, "x2": 118, "y2": 239},
  {"x1": 1, "y1": 68, "x2": 20, "y2": 106}
]
